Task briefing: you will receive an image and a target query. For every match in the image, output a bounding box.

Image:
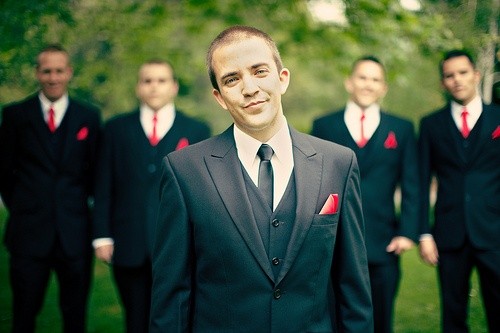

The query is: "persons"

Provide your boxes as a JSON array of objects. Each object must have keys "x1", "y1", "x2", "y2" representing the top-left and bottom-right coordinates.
[
  {"x1": 90, "y1": 55, "x2": 212, "y2": 332},
  {"x1": 310, "y1": 55, "x2": 415, "y2": 332},
  {"x1": 416, "y1": 50, "x2": 500, "y2": 332},
  {"x1": 147, "y1": 24, "x2": 375, "y2": 332},
  {"x1": 0, "y1": 44, "x2": 103, "y2": 333}
]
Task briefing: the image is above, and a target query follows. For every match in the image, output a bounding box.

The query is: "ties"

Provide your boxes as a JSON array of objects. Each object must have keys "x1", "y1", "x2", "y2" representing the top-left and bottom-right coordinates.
[
  {"x1": 150, "y1": 114, "x2": 159, "y2": 141},
  {"x1": 459, "y1": 109, "x2": 470, "y2": 139},
  {"x1": 47, "y1": 106, "x2": 57, "y2": 132},
  {"x1": 257, "y1": 143, "x2": 276, "y2": 211},
  {"x1": 358, "y1": 114, "x2": 368, "y2": 150}
]
